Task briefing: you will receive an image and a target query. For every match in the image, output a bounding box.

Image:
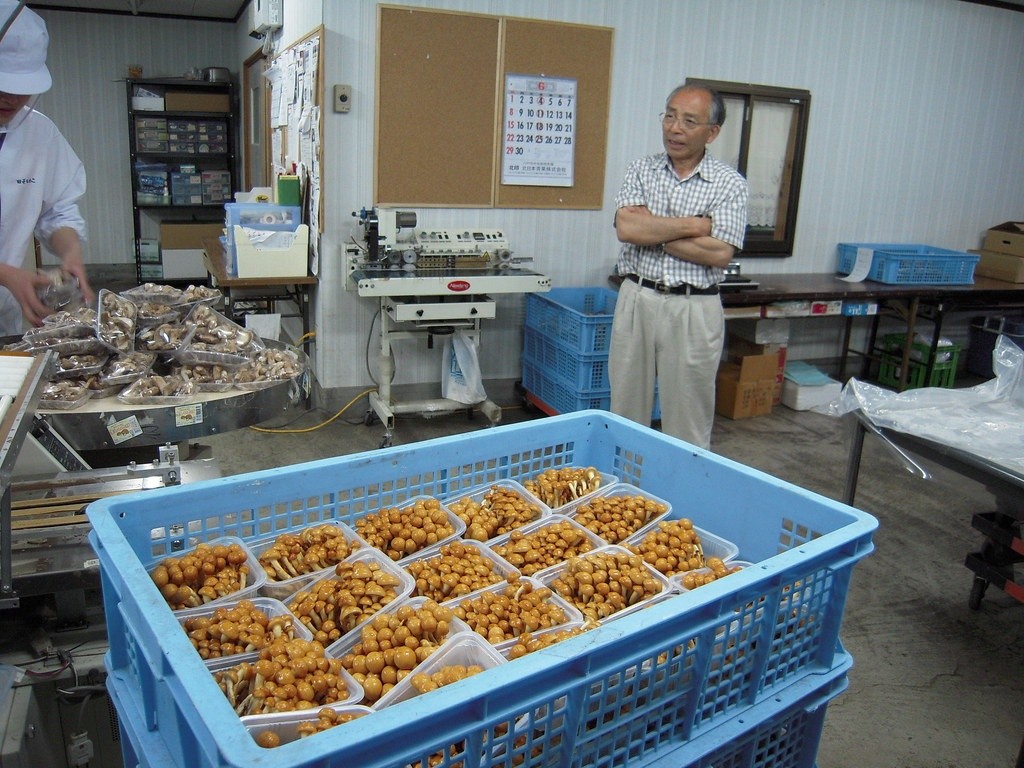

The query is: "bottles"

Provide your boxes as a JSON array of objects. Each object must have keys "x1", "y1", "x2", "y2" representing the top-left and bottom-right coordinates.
[{"x1": 188, "y1": 66, "x2": 204, "y2": 80}]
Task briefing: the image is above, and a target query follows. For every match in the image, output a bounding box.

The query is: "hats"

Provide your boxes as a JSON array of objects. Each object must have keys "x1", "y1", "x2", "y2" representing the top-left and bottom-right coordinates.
[{"x1": 0, "y1": 1, "x2": 53, "y2": 97}]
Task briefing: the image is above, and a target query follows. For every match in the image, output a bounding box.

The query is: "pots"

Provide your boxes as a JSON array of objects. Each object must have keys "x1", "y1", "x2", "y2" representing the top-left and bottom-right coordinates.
[{"x1": 204, "y1": 67, "x2": 230, "y2": 83}]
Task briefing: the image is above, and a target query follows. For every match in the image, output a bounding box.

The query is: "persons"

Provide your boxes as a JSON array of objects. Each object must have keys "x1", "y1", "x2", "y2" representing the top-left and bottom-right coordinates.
[
  {"x1": 607, "y1": 82, "x2": 749, "y2": 490},
  {"x1": 0, "y1": 0, "x2": 95, "y2": 337}
]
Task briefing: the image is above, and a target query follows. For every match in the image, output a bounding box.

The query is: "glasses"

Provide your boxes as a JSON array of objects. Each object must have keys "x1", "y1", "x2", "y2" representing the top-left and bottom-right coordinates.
[{"x1": 657, "y1": 112, "x2": 720, "y2": 129}]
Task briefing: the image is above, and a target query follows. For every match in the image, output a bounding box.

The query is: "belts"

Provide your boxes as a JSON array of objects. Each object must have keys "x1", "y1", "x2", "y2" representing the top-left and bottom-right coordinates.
[{"x1": 627, "y1": 274, "x2": 719, "y2": 296}]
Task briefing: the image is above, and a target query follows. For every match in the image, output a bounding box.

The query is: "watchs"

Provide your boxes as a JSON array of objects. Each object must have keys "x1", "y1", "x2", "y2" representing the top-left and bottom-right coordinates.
[{"x1": 655, "y1": 242, "x2": 665, "y2": 254}]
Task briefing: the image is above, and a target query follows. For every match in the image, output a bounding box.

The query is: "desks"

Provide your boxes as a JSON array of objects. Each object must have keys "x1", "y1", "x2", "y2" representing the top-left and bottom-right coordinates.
[
  {"x1": 842, "y1": 383, "x2": 1024, "y2": 611},
  {"x1": 718, "y1": 274, "x2": 934, "y2": 387},
  {"x1": 921, "y1": 275, "x2": 1024, "y2": 387},
  {"x1": 200, "y1": 235, "x2": 319, "y2": 361},
  {"x1": 0, "y1": 330, "x2": 311, "y2": 462}
]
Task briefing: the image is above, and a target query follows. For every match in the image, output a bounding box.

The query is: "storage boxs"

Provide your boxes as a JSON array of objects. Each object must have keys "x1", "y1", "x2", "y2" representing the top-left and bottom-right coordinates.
[
  {"x1": 160, "y1": 249, "x2": 208, "y2": 280},
  {"x1": 131, "y1": 97, "x2": 164, "y2": 111},
  {"x1": 159, "y1": 219, "x2": 225, "y2": 248},
  {"x1": 982, "y1": 220, "x2": 1024, "y2": 257},
  {"x1": 179, "y1": 162, "x2": 195, "y2": 174},
  {"x1": 524, "y1": 286, "x2": 621, "y2": 356},
  {"x1": 165, "y1": 93, "x2": 231, "y2": 113},
  {"x1": 136, "y1": 262, "x2": 161, "y2": 277},
  {"x1": 522, "y1": 363, "x2": 662, "y2": 420},
  {"x1": 522, "y1": 325, "x2": 658, "y2": 394},
  {"x1": 877, "y1": 332, "x2": 962, "y2": 389},
  {"x1": 226, "y1": 201, "x2": 307, "y2": 279},
  {"x1": 729, "y1": 338, "x2": 788, "y2": 407},
  {"x1": 782, "y1": 362, "x2": 843, "y2": 411},
  {"x1": 135, "y1": 161, "x2": 171, "y2": 205},
  {"x1": 728, "y1": 318, "x2": 792, "y2": 345},
  {"x1": 279, "y1": 174, "x2": 302, "y2": 206},
  {"x1": 134, "y1": 117, "x2": 229, "y2": 154},
  {"x1": 201, "y1": 170, "x2": 232, "y2": 206},
  {"x1": 133, "y1": 236, "x2": 159, "y2": 263},
  {"x1": 969, "y1": 315, "x2": 1024, "y2": 375},
  {"x1": 966, "y1": 249, "x2": 1024, "y2": 284},
  {"x1": 836, "y1": 243, "x2": 979, "y2": 284},
  {"x1": 172, "y1": 171, "x2": 203, "y2": 206},
  {"x1": 86, "y1": 410, "x2": 881, "y2": 768},
  {"x1": 714, "y1": 353, "x2": 779, "y2": 421}
]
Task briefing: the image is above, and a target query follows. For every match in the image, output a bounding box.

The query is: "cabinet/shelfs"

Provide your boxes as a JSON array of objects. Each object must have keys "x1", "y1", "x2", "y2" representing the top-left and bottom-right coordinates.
[{"x1": 126, "y1": 77, "x2": 241, "y2": 288}]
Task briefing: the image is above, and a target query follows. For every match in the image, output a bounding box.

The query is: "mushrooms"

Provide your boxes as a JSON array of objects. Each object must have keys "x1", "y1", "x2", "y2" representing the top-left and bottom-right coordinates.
[
  {"x1": 24, "y1": 283, "x2": 302, "y2": 409},
  {"x1": 150, "y1": 466, "x2": 814, "y2": 768}
]
